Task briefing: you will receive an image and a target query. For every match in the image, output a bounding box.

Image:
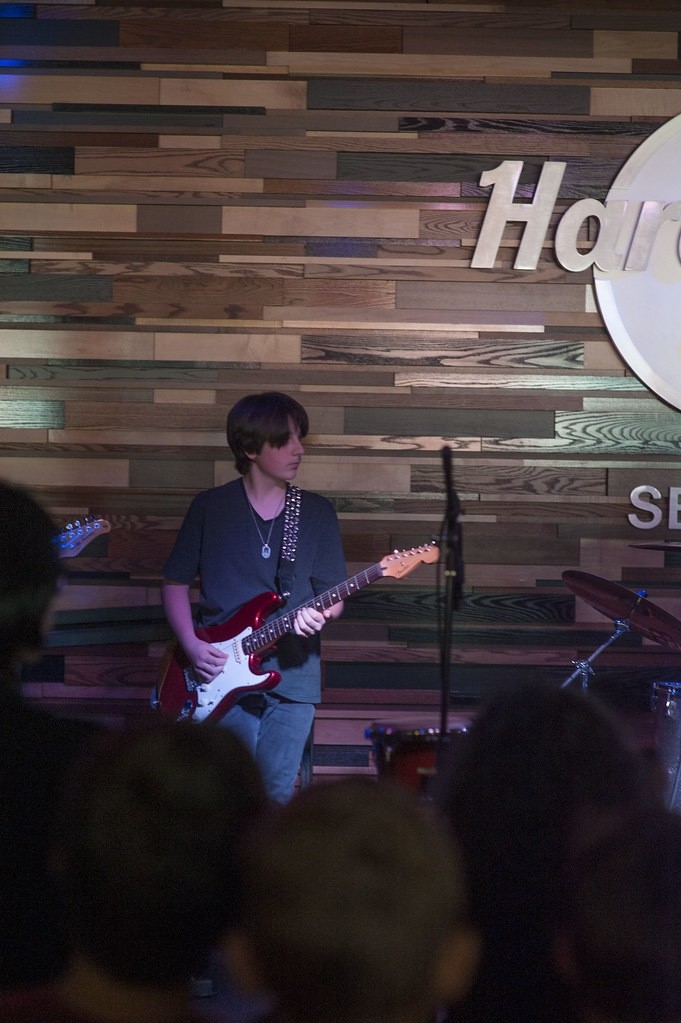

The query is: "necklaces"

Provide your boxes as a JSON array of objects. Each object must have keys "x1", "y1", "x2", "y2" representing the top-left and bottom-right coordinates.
[{"x1": 241, "y1": 478, "x2": 286, "y2": 558}]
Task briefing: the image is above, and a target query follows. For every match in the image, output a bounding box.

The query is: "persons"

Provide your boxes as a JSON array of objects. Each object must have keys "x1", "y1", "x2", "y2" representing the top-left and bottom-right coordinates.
[
  {"x1": 0, "y1": 473, "x2": 272, "y2": 1023},
  {"x1": 220, "y1": 775, "x2": 480, "y2": 1023},
  {"x1": 162, "y1": 394, "x2": 346, "y2": 803},
  {"x1": 437, "y1": 669, "x2": 681, "y2": 1023}
]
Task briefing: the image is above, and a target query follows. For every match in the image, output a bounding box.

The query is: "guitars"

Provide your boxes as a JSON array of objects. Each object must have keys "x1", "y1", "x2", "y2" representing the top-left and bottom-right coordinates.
[
  {"x1": 52, "y1": 514, "x2": 111, "y2": 559},
  {"x1": 152, "y1": 542, "x2": 440, "y2": 727}
]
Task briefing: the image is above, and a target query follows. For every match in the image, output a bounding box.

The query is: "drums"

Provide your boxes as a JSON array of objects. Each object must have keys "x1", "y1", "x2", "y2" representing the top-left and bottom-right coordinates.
[
  {"x1": 650, "y1": 676, "x2": 681, "y2": 746},
  {"x1": 367, "y1": 715, "x2": 473, "y2": 794}
]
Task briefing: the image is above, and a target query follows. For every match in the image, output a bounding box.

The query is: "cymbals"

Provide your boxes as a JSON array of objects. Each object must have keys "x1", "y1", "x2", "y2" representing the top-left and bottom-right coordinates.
[{"x1": 561, "y1": 570, "x2": 681, "y2": 651}]
